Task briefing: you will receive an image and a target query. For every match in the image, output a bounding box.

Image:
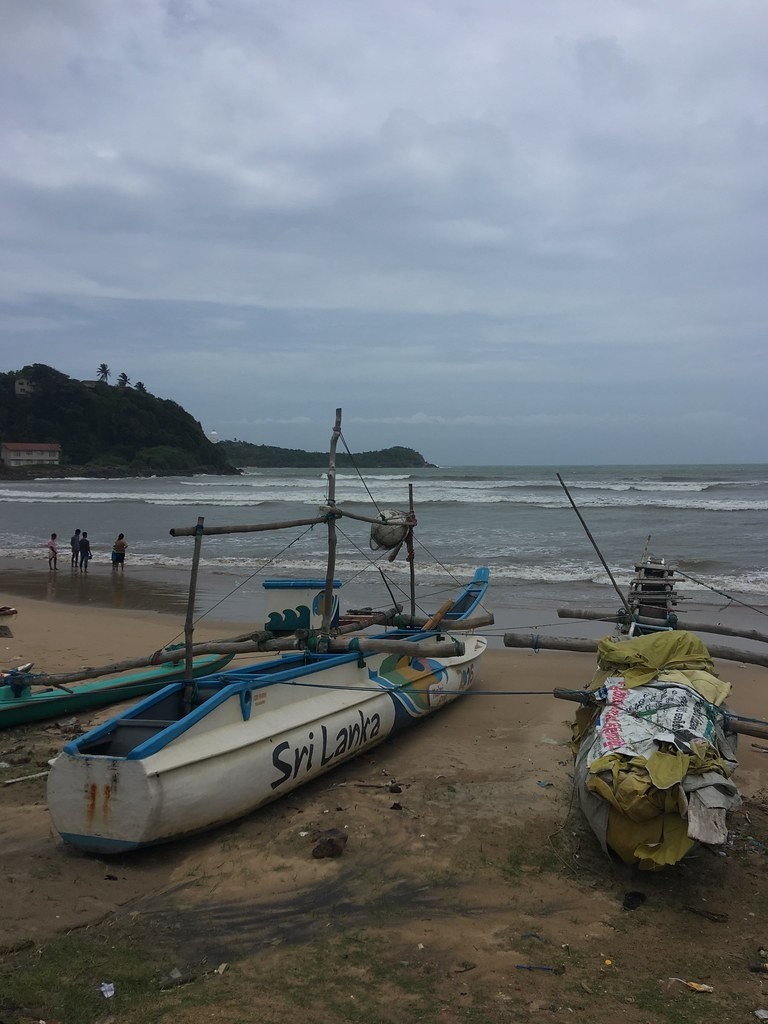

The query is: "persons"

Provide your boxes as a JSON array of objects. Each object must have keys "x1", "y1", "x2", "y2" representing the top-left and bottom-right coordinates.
[
  {"x1": 79, "y1": 532, "x2": 92, "y2": 573},
  {"x1": 47, "y1": 533, "x2": 61, "y2": 572},
  {"x1": 70, "y1": 529, "x2": 81, "y2": 568},
  {"x1": 111, "y1": 544, "x2": 117, "y2": 570},
  {"x1": 114, "y1": 533, "x2": 128, "y2": 570}
]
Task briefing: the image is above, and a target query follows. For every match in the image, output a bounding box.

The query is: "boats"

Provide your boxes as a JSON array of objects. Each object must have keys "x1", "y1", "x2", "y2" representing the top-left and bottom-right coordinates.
[
  {"x1": 0, "y1": 648, "x2": 235, "y2": 729},
  {"x1": 568, "y1": 533, "x2": 737, "y2": 877},
  {"x1": 44, "y1": 407, "x2": 496, "y2": 860},
  {"x1": 262, "y1": 578, "x2": 342, "y2": 590}
]
